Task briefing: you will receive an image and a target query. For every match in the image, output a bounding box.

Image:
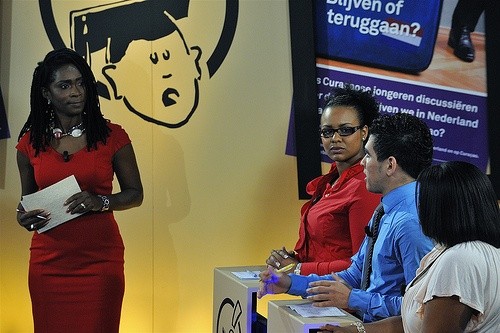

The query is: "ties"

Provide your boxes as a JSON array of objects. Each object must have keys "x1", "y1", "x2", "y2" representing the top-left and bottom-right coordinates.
[{"x1": 362, "y1": 206, "x2": 384, "y2": 292}]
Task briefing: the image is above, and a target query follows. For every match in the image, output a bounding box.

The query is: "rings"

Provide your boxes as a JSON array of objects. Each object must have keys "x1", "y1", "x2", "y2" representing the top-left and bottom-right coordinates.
[
  {"x1": 81, "y1": 203, "x2": 86, "y2": 208},
  {"x1": 31, "y1": 224, "x2": 33, "y2": 229}
]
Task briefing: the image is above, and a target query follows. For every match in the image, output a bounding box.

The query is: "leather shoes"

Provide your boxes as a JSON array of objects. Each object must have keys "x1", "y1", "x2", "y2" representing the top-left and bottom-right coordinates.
[{"x1": 448, "y1": 20, "x2": 475, "y2": 62}]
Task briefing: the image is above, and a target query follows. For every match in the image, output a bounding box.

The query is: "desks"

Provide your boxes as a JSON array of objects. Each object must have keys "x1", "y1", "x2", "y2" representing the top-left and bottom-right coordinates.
[{"x1": 213, "y1": 265, "x2": 363, "y2": 333}]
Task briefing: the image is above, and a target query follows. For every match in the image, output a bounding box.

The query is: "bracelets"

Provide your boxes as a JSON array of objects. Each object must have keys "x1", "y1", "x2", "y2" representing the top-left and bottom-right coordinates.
[{"x1": 98, "y1": 195, "x2": 110, "y2": 212}]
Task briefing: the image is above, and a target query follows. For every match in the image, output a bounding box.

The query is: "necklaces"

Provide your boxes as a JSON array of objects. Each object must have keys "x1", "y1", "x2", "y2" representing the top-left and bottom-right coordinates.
[
  {"x1": 50, "y1": 123, "x2": 85, "y2": 138},
  {"x1": 409, "y1": 247, "x2": 449, "y2": 287}
]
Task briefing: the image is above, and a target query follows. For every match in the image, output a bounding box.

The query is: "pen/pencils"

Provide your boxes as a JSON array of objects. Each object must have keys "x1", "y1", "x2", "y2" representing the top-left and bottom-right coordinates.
[
  {"x1": 257, "y1": 263, "x2": 296, "y2": 283},
  {"x1": 16, "y1": 207, "x2": 48, "y2": 220}
]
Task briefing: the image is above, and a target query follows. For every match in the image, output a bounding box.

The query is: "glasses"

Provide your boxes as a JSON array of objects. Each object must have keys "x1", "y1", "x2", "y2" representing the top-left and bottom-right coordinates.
[{"x1": 318, "y1": 124, "x2": 364, "y2": 138}]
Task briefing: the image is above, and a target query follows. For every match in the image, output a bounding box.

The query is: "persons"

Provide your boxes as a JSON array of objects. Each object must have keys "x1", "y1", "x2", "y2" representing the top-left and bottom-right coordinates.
[
  {"x1": 267, "y1": 86, "x2": 384, "y2": 277},
  {"x1": 318, "y1": 160, "x2": 500, "y2": 333},
  {"x1": 257, "y1": 112, "x2": 438, "y2": 322},
  {"x1": 447, "y1": 0, "x2": 487, "y2": 63},
  {"x1": 15, "y1": 48, "x2": 144, "y2": 333}
]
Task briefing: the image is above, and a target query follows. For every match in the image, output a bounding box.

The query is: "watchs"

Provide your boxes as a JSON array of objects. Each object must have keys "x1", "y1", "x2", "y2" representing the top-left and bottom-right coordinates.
[
  {"x1": 294, "y1": 263, "x2": 302, "y2": 275},
  {"x1": 351, "y1": 320, "x2": 366, "y2": 333}
]
